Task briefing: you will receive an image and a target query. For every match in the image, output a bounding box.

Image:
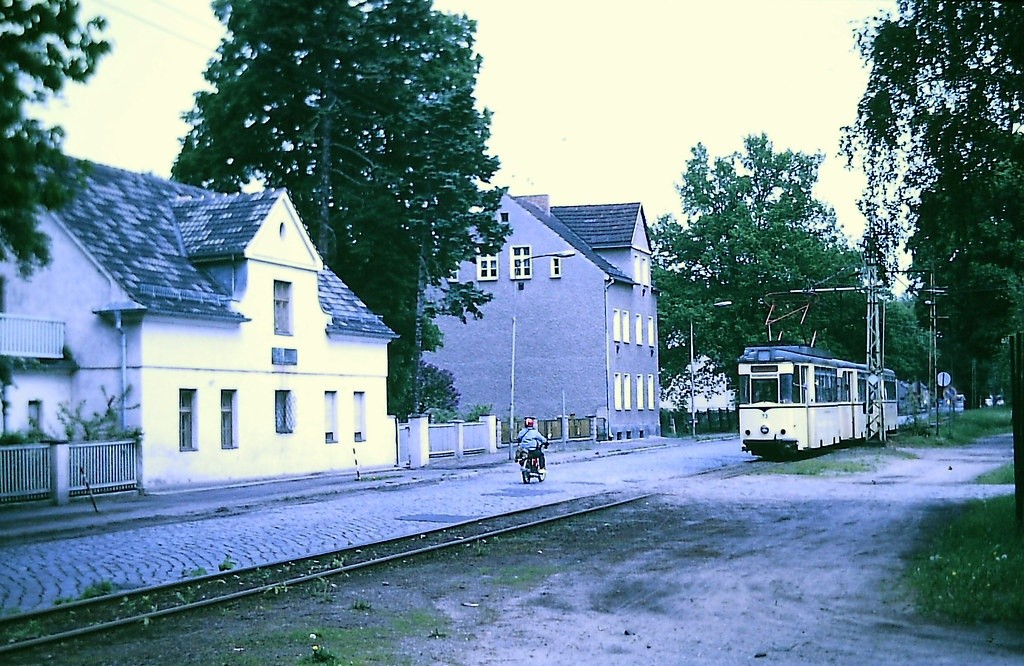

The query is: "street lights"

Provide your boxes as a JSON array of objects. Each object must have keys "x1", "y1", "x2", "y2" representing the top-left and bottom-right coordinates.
[
  {"x1": 690, "y1": 301, "x2": 732, "y2": 439},
  {"x1": 507, "y1": 249, "x2": 577, "y2": 461}
]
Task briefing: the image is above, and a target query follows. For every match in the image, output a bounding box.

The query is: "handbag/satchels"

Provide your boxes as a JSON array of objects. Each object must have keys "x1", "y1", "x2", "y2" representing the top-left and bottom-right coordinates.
[{"x1": 518, "y1": 429, "x2": 531, "y2": 446}]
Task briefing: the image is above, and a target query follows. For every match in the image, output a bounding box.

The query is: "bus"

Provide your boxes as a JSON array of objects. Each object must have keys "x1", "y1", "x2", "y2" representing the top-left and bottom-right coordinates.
[{"x1": 737, "y1": 342, "x2": 900, "y2": 463}]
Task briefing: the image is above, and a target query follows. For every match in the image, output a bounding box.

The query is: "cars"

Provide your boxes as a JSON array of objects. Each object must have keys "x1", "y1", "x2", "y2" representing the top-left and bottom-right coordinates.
[{"x1": 947, "y1": 393, "x2": 1006, "y2": 408}]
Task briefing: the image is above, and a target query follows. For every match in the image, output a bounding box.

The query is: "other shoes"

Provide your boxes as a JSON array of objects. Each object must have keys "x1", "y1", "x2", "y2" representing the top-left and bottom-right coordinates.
[{"x1": 539, "y1": 468, "x2": 546, "y2": 473}]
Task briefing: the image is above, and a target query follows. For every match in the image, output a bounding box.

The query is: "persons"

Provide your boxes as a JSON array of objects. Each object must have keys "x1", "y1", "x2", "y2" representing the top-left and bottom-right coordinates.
[{"x1": 515, "y1": 417, "x2": 549, "y2": 473}]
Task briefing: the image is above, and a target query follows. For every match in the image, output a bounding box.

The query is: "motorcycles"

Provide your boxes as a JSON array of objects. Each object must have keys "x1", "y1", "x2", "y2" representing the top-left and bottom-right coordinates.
[{"x1": 519, "y1": 434, "x2": 552, "y2": 485}]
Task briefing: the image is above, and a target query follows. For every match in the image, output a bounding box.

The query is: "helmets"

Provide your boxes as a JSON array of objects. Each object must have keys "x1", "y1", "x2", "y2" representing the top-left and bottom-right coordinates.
[{"x1": 525, "y1": 418, "x2": 533, "y2": 428}]
